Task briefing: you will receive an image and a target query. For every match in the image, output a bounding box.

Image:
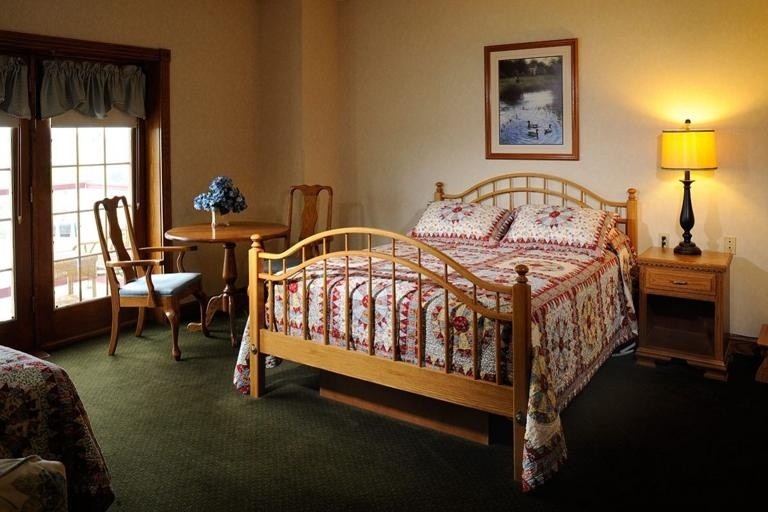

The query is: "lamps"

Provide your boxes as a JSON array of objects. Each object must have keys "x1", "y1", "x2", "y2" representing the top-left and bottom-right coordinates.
[{"x1": 660, "y1": 118, "x2": 719, "y2": 254}]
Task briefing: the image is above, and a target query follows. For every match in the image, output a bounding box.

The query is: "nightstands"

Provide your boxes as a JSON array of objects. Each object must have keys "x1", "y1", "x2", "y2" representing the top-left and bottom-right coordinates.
[{"x1": 635, "y1": 242, "x2": 734, "y2": 382}]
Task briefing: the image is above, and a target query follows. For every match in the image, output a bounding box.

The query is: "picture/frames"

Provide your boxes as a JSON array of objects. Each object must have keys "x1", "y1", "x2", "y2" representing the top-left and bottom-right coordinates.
[{"x1": 484, "y1": 37, "x2": 578, "y2": 160}]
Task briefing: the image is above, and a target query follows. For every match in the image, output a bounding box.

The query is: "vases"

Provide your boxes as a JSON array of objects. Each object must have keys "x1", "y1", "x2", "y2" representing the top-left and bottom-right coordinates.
[{"x1": 210, "y1": 208, "x2": 231, "y2": 229}]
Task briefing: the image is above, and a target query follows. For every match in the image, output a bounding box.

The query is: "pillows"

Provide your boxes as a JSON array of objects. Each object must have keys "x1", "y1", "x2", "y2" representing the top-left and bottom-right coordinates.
[
  {"x1": 500, "y1": 204, "x2": 619, "y2": 261},
  {"x1": 408, "y1": 200, "x2": 513, "y2": 251}
]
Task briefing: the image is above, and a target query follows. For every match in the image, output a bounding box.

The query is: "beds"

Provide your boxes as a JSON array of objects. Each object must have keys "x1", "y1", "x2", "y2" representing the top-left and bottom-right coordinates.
[{"x1": 1, "y1": 340, "x2": 117, "y2": 511}]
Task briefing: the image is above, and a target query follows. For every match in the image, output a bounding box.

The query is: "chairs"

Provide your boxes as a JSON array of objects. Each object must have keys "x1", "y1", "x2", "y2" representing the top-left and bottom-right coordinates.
[
  {"x1": 248, "y1": 171, "x2": 637, "y2": 488},
  {"x1": 94, "y1": 196, "x2": 210, "y2": 361},
  {"x1": 285, "y1": 184, "x2": 333, "y2": 263}
]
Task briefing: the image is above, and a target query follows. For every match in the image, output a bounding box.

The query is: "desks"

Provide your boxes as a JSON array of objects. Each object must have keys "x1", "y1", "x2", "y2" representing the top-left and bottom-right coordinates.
[{"x1": 164, "y1": 222, "x2": 289, "y2": 346}]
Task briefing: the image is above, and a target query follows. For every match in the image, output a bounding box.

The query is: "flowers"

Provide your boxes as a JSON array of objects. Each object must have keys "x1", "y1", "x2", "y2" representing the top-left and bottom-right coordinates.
[{"x1": 195, "y1": 176, "x2": 246, "y2": 214}]
